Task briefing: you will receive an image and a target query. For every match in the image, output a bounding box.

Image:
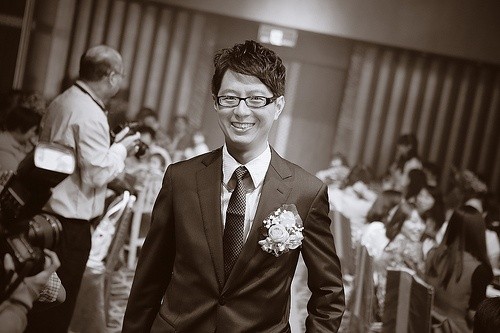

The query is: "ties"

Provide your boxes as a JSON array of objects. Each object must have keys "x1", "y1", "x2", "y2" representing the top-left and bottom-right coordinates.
[{"x1": 223, "y1": 166, "x2": 249, "y2": 285}]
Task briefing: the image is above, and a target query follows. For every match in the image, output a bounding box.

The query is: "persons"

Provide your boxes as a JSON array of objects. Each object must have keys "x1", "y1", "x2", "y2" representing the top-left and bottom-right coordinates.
[
  {"x1": 422, "y1": 205, "x2": 493, "y2": 331},
  {"x1": 0, "y1": 87, "x2": 45, "y2": 190},
  {"x1": 326, "y1": 134, "x2": 500, "y2": 322},
  {"x1": 28, "y1": 44, "x2": 141, "y2": 333},
  {"x1": 1, "y1": 247, "x2": 69, "y2": 333},
  {"x1": 120, "y1": 39, "x2": 345, "y2": 333},
  {"x1": 107, "y1": 89, "x2": 210, "y2": 160}
]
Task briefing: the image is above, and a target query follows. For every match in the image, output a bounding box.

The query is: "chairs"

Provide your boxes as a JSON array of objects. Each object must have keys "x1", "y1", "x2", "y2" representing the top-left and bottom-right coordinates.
[
  {"x1": 68, "y1": 195, "x2": 136, "y2": 333},
  {"x1": 331, "y1": 209, "x2": 433, "y2": 333}
]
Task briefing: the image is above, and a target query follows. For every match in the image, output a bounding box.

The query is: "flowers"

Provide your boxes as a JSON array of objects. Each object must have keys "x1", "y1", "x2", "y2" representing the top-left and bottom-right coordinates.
[{"x1": 258, "y1": 203, "x2": 305, "y2": 257}]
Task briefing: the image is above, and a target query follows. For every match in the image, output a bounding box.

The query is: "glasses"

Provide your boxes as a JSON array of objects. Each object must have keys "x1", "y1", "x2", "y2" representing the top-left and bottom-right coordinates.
[{"x1": 216, "y1": 96, "x2": 279, "y2": 108}]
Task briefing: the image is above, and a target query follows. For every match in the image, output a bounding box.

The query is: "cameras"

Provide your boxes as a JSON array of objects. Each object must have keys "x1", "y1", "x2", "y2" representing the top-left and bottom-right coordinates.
[
  {"x1": 123, "y1": 123, "x2": 157, "y2": 160},
  {"x1": 0, "y1": 140, "x2": 79, "y2": 278}
]
